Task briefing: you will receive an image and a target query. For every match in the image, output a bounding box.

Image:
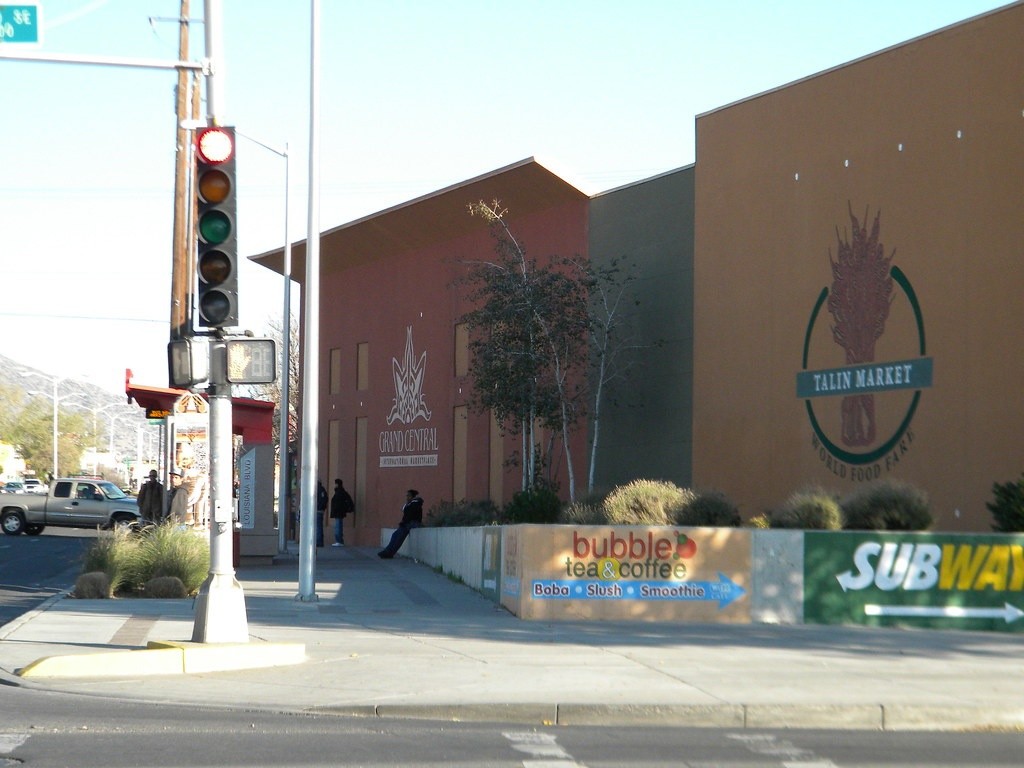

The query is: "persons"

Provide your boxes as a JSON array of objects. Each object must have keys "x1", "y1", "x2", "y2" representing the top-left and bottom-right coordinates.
[
  {"x1": 330, "y1": 479, "x2": 354, "y2": 546},
  {"x1": 185, "y1": 463, "x2": 206, "y2": 526},
  {"x1": 377, "y1": 489, "x2": 424, "y2": 559},
  {"x1": 91, "y1": 487, "x2": 99, "y2": 494},
  {"x1": 137, "y1": 469, "x2": 163, "y2": 526},
  {"x1": 166, "y1": 466, "x2": 189, "y2": 528},
  {"x1": 316, "y1": 480, "x2": 328, "y2": 547}
]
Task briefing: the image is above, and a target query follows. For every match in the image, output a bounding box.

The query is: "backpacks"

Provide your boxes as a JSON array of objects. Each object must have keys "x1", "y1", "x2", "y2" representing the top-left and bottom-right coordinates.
[{"x1": 340, "y1": 491, "x2": 354, "y2": 513}]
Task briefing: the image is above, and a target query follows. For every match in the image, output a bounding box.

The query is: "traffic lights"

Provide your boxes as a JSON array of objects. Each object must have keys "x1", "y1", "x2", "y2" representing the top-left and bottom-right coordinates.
[
  {"x1": 224, "y1": 339, "x2": 278, "y2": 386},
  {"x1": 193, "y1": 126, "x2": 238, "y2": 327},
  {"x1": 167, "y1": 340, "x2": 210, "y2": 385}
]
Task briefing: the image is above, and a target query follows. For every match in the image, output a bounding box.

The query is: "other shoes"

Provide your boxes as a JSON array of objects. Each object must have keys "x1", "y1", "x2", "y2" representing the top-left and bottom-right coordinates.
[
  {"x1": 332, "y1": 542, "x2": 345, "y2": 547},
  {"x1": 378, "y1": 551, "x2": 393, "y2": 559}
]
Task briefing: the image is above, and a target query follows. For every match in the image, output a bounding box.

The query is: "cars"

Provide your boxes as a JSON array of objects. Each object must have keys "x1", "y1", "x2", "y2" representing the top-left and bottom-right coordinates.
[{"x1": 0, "y1": 475, "x2": 133, "y2": 495}]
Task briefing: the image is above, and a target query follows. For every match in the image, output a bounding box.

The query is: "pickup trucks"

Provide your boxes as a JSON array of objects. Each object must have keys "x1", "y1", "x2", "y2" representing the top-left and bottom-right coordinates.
[{"x1": 0, "y1": 477, "x2": 143, "y2": 536}]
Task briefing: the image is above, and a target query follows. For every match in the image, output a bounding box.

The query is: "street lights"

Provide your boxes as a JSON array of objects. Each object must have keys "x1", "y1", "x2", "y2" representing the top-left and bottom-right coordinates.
[
  {"x1": 95, "y1": 400, "x2": 137, "y2": 468},
  {"x1": 60, "y1": 402, "x2": 129, "y2": 478},
  {"x1": 181, "y1": 117, "x2": 293, "y2": 555},
  {"x1": 18, "y1": 369, "x2": 80, "y2": 478}
]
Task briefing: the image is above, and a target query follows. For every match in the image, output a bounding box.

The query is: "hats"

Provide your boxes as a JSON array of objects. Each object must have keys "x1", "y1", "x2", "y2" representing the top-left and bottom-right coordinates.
[{"x1": 169, "y1": 467, "x2": 184, "y2": 478}]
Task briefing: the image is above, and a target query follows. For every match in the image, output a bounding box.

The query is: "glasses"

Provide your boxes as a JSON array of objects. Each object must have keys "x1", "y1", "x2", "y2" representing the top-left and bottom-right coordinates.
[{"x1": 149, "y1": 475, "x2": 155, "y2": 477}]
